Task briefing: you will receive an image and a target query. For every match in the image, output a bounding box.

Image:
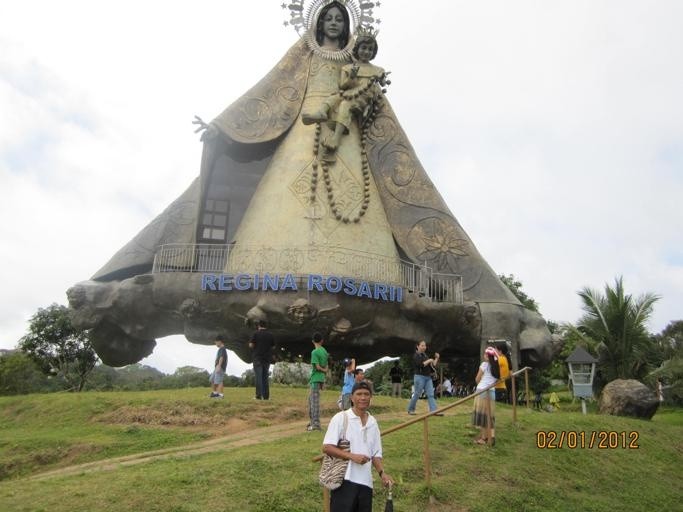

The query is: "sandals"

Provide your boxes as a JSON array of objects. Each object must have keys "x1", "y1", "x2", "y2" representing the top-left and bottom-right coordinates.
[{"x1": 474, "y1": 438, "x2": 495, "y2": 446}]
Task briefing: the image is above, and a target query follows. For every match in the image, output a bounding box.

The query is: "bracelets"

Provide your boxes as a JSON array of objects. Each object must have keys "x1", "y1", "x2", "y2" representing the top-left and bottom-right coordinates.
[{"x1": 378, "y1": 470, "x2": 384, "y2": 478}]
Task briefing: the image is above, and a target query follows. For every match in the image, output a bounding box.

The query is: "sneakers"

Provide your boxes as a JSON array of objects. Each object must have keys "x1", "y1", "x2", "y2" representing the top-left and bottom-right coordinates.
[
  {"x1": 306, "y1": 426, "x2": 320, "y2": 430},
  {"x1": 408, "y1": 412, "x2": 416, "y2": 415},
  {"x1": 435, "y1": 412, "x2": 444, "y2": 416},
  {"x1": 207, "y1": 392, "x2": 224, "y2": 399}
]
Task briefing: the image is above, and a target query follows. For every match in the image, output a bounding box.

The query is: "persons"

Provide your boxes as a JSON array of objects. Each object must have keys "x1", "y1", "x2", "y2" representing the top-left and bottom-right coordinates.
[
  {"x1": 492, "y1": 341, "x2": 511, "y2": 400},
  {"x1": 407, "y1": 338, "x2": 445, "y2": 416},
  {"x1": 342, "y1": 357, "x2": 356, "y2": 411},
  {"x1": 389, "y1": 359, "x2": 404, "y2": 398},
  {"x1": 194, "y1": 0, "x2": 405, "y2": 288},
  {"x1": 208, "y1": 333, "x2": 226, "y2": 400},
  {"x1": 303, "y1": 331, "x2": 329, "y2": 431},
  {"x1": 471, "y1": 347, "x2": 501, "y2": 447},
  {"x1": 180, "y1": 298, "x2": 201, "y2": 320},
  {"x1": 317, "y1": 380, "x2": 399, "y2": 512},
  {"x1": 247, "y1": 318, "x2": 275, "y2": 402},
  {"x1": 331, "y1": 319, "x2": 353, "y2": 342},
  {"x1": 66, "y1": 286, "x2": 86, "y2": 308},
  {"x1": 300, "y1": 29, "x2": 390, "y2": 152},
  {"x1": 337, "y1": 369, "x2": 373, "y2": 411},
  {"x1": 436, "y1": 374, "x2": 561, "y2": 410},
  {"x1": 285, "y1": 299, "x2": 318, "y2": 327}
]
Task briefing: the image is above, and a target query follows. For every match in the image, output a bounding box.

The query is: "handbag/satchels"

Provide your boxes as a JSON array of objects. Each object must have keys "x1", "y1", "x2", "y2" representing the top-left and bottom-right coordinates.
[{"x1": 318, "y1": 440, "x2": 349, "y2": 491}]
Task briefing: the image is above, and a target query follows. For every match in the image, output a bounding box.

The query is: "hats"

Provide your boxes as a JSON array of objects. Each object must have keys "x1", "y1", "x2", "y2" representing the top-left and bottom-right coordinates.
[
  {"x1": 342, "y1": 358, "x2": 352, "y2": 366},
  {"x1": 485, "y1": 348, "x2": 494, "y2": 356},
  {"x1": 351, "y1": 383, "x2": 371, "y2": 407}
]
